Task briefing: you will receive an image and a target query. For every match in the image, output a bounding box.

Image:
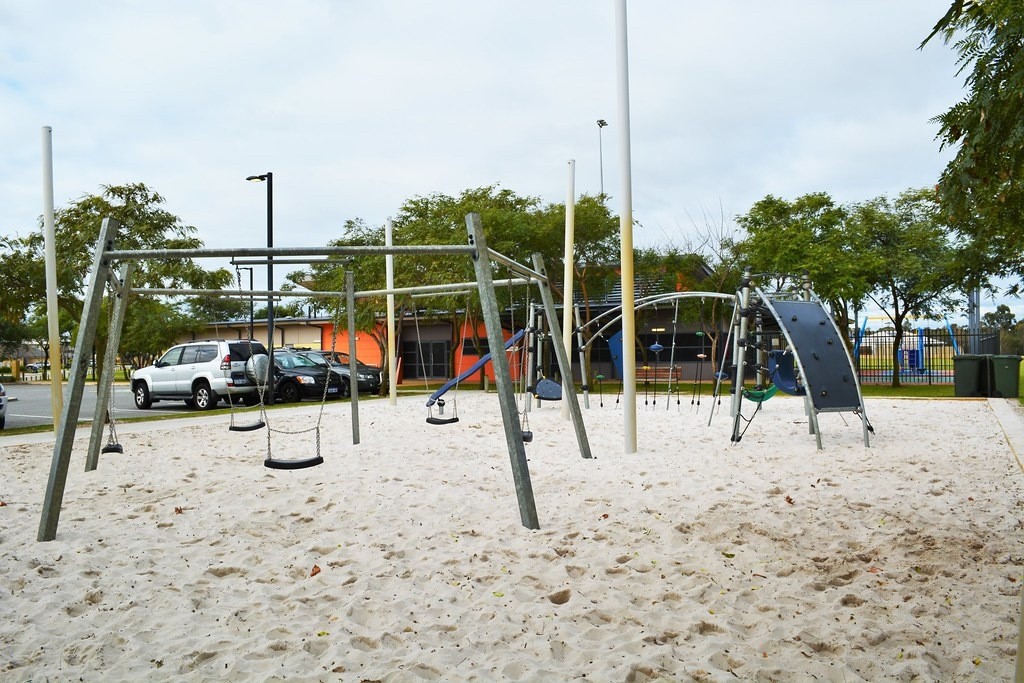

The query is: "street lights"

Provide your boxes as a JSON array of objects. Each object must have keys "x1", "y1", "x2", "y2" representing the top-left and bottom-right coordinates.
[
  {"x1": 596, "y1": 119, "x2": 609, "y2": 201},
  {"x1": 245, "y1": 174, "x2": 272, "y2": 404}
]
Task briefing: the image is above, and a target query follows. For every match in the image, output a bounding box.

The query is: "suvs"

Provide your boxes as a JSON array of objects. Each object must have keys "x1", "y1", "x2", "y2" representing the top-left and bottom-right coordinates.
[
  {"x1": 131, "y1": 339, "x2": 271, "y2": 409},
  {"x1": 27, "y1": 361, "x2": 49, "y2": 370}
]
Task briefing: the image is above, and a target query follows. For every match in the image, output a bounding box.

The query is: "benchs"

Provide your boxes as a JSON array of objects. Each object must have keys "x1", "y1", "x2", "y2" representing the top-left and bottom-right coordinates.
[{"x1": 636, "y1": 366, "x2": 683, "y2": 382}]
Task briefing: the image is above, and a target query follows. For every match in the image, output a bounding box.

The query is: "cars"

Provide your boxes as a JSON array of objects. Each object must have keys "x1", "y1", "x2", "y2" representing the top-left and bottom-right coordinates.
[
  {"x1": 254, "y1": 351, "x2": 341, "y2": 403},
  {"x1": 282, "y1": 351, "x2": 382, "y2": 395},
  {"x1": 0, "y1": 383, "x2": 7, "y2": 430},
  {"x1": 90, "y1": 359, "x2": 96, "y2": 367}
]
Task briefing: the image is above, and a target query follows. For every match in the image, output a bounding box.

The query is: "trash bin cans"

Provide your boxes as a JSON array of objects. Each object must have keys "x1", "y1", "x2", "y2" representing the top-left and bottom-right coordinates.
[
  {"x1": 951, "y1": 354, "x2": 992, "y2": 397},
  {"x1": 984, "y1": 354, "x2": 1024, "y2": 399}
]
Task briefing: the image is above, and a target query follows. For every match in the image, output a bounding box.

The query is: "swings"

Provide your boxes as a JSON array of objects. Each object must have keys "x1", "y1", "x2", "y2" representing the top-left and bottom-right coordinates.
[
  {"x1": 411, "y1": 254, "x2": 471, "y2": 426},
  {"x1": 505, "y1": 267, "x2": 534, "y2": 443},
  {"x1": 100, "y1": 273, "x2": 124, "y2": 454},
  {"x1": 210, "y1": 264, "x2": 348, "y2": 471}
]
